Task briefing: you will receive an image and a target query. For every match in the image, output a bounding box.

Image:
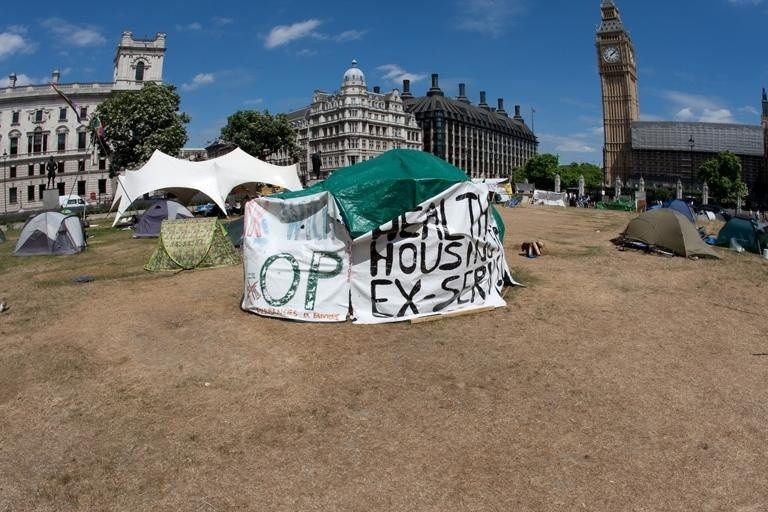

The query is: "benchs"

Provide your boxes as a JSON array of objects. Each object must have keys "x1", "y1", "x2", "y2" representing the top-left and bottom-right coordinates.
[
  {"x1": 94, "y1": 116, "x2": 105, "y2": 138},
  {"x1": 49, "y1": 82, "x2": 82, "y2": 124}
]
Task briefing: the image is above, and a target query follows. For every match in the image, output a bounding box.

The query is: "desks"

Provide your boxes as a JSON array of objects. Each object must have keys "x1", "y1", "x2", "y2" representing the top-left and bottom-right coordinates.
[
  {"x1": 2, "y1": 149, "x2": 9, "y2": 218},
  {"x1": 687, "y1": 134, "x2": 696, "y2": 205}
]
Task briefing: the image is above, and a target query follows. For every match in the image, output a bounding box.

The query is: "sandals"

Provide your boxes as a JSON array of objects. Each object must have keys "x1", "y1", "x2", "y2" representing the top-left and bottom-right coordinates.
[{"x1": 566, "y1": 188, "x2": 588, "y2": 194}]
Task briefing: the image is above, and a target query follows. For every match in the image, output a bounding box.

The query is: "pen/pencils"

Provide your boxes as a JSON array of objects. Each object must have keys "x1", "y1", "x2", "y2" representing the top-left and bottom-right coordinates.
[{"x1": 62, "y1": 198, "x2": 89, "y2": 208}]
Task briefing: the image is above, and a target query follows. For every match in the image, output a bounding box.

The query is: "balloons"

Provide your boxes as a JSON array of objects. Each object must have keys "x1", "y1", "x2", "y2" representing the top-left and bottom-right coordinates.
[{"x1": 602, "y1": 45, "x2": 620, "y2": 64}]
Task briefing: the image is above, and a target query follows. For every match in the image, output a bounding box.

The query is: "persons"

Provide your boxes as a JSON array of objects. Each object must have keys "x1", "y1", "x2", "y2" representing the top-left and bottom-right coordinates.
[
  {"x1": 46, "y1": 156, "x2": 57, "y2": 189},
  {"x1": 132, "y1": 215, "x2": 139, "y2": 225}
]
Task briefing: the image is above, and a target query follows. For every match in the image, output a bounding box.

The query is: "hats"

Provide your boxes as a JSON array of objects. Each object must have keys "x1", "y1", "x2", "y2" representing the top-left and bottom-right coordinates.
[
  {"x1": 734, "y1": 214, "x2": 758, "y2": 226},
  {"x1": 694, "y1": 204, "x2": 731, "y2": 221},
  {"x1": 197, "y1": 203, "x2": 229, "y2": 215}
]
